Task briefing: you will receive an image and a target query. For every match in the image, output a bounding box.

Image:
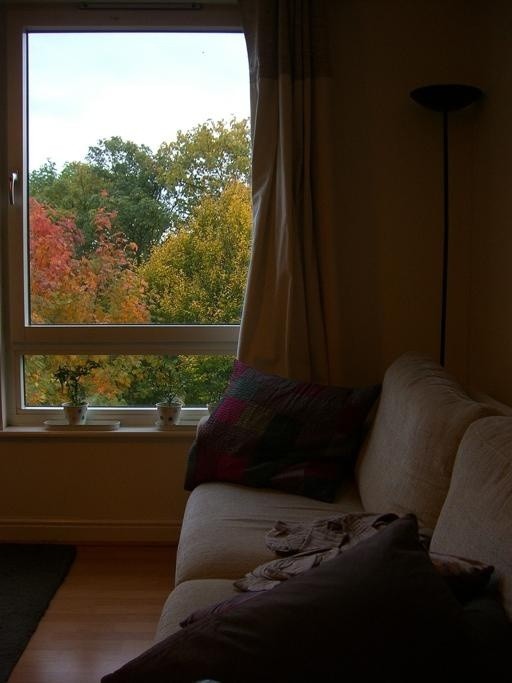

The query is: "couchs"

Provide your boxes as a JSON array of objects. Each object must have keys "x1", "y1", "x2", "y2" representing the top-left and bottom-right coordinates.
[{"x1": 151, "y1": 351, "x2": 510, "y2": 683}]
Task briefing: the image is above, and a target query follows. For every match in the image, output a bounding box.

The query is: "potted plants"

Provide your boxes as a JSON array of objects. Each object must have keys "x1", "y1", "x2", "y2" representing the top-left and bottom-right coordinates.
[
  {"x1": 153, "y1": 357, "x2": 191, "y2": 426},
  {"x1": 53, "y1": 359, "x2": 105, "y2": 425}
]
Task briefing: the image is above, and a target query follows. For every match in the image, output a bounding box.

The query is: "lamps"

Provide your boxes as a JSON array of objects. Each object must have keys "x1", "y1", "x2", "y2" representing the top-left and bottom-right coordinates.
[{"x1": 411, "y1": 80, "x2": 484, "y2": 367}]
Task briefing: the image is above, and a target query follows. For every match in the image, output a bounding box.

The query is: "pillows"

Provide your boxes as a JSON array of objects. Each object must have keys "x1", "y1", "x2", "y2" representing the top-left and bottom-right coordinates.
[
  {"x1": 100, "y1": 512, "x2": 493, "y2": 683},
  {"x1": 186, "y1": 361, "x2": 383, "y2": 503}
]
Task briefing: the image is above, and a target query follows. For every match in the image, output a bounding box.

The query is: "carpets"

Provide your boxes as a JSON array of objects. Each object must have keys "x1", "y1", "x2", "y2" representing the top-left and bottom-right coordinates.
[{"x1": 2, "y1": 539, "x2": 77, "y2": 682}]
